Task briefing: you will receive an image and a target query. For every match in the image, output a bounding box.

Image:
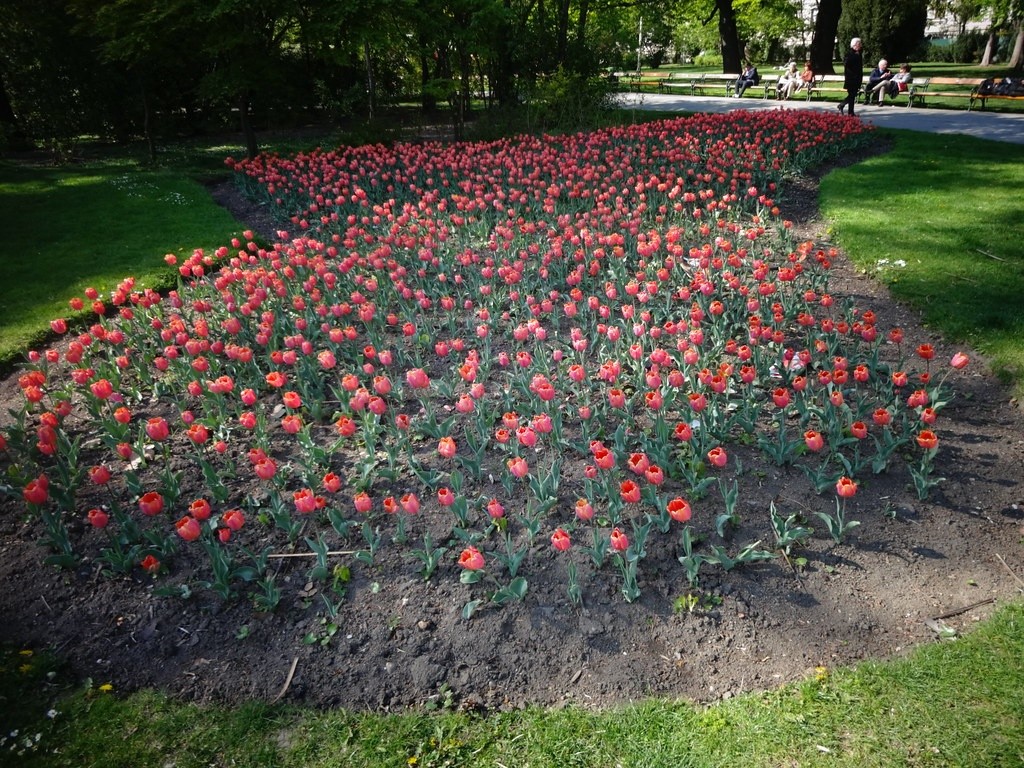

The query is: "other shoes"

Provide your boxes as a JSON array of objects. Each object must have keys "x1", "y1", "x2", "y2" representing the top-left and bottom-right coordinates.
[
  {"x1": 863, "y1": 100, "x2": 869, "y2": 105},
  {"x1": 866, "y1": 90, "x2": 872, "y2": 96},
  {"x1": 733, "y1": 93, "x2": 740, "y2": 98},
  {"x1": 837, "y1": 105, "x2": 843, "y2": 115},
  {"x1": 776, "y1": 89, "x2": 783, "y2": 95},
  {"x1": 880, "y1": 102, "x2": 883, "y2": 107}
]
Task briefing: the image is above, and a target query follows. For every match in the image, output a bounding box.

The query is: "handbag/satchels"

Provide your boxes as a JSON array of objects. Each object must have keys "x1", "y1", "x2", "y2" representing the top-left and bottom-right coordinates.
[
  {"x1": 737, "y1": 74, "x2": 745, "y2": 88},
  {"x1": 978, "y1": 78, "x2": 1024, "y2": 97},
  {"x1": 898, "y1": 83, "x2": 907, "y2": 92}
]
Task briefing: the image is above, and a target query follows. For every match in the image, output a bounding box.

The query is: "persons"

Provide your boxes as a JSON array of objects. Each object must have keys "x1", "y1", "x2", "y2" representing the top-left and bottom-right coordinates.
[
  {"x1": 837, "y1": 38, "x2": 862, "y2": 117},
  {"x1": 729, "y1": 60, "x2": 911, "y2": 108}
]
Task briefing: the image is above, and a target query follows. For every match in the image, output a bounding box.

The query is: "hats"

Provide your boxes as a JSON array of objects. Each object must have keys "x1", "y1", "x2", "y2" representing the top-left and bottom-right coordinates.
[{"x1": 744, "y1": 62, "x2": 752, "y2": 67}]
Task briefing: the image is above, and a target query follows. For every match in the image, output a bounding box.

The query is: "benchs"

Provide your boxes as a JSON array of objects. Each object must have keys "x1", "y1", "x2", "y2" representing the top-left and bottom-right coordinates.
[{"x1": 599, "y1": 71, "x2": 1024, "y2": 112}]
